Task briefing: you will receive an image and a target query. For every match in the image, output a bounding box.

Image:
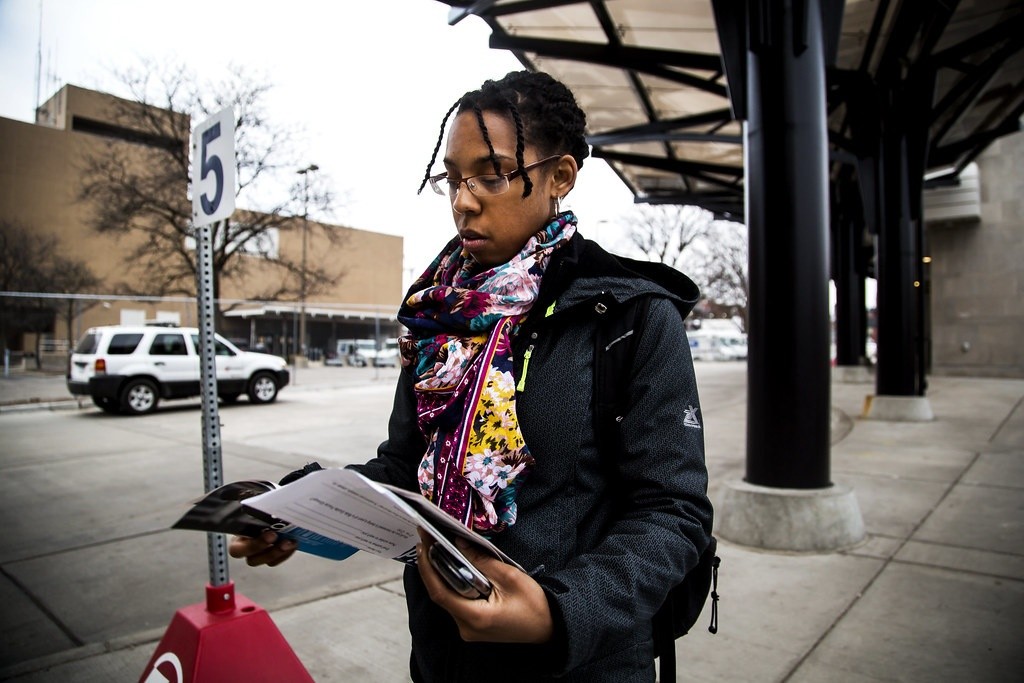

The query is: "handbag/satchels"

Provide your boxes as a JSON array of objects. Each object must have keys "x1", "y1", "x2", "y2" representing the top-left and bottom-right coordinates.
[{"x1": 594, "y1": 292, "x2": 721, "y2": 683}]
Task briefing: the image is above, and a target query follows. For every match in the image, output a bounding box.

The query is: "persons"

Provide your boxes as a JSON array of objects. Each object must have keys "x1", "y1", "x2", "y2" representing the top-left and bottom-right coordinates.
[{"x1": 230, "y1": 70, "x2": 715, "y2": 683}]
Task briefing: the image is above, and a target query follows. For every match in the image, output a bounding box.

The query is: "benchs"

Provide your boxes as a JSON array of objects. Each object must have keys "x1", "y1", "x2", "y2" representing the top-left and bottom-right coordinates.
[{"x1": 39, "y1": 338, "x2": 80, "y2": 361}]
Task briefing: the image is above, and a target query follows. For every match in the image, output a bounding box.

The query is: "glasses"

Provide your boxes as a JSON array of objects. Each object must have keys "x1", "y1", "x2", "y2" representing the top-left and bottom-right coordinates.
[{"x1": 429, "y1": 155, "x2": 563, "y2": 197}]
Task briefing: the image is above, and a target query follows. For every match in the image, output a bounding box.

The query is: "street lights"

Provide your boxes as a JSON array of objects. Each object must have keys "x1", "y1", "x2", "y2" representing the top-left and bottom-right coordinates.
[{"x1": 296, "y1": 165, "x2": 319, "y2": 370}]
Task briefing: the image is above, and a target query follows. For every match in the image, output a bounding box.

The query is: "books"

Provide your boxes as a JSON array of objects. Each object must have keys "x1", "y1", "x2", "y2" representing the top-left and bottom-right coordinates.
[{"x1": 171, "y1": 480, "x2": 528, "y2": 575}]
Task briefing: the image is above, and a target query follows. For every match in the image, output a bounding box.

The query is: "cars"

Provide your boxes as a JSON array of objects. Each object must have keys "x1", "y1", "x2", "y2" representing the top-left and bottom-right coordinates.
[
  {"x1": 689, "y1": 317, "x2": 746, "y2": 360},
  {"x1": 328, "y1": 338, "x2": 401, "y2": 366}
]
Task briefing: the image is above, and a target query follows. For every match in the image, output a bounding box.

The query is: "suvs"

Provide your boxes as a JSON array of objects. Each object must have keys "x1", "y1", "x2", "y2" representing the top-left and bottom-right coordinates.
[{"x1": 66, "y1": 321, "x2": 291, "y2": 416}]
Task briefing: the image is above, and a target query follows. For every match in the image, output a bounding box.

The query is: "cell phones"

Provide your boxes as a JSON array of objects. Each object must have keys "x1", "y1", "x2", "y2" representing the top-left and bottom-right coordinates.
[{"x1": 427, "y1": 542, "x2": 492, "y2": 600}]
